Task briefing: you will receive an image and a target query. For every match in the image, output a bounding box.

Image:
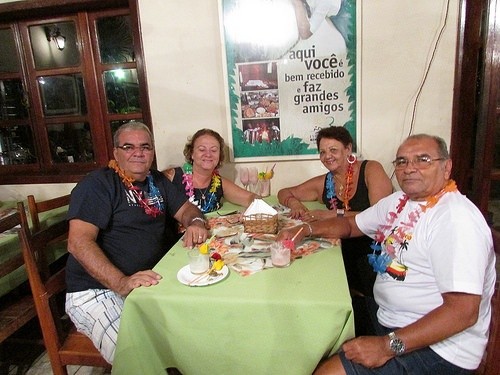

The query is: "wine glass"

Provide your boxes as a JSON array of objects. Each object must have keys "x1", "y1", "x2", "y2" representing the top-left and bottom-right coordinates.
[{"x1": 239, "y1": 167, "x2": 257, "y2": 193}]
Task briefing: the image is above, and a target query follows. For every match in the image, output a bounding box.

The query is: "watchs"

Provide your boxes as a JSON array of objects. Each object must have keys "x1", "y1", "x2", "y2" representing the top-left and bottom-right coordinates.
[{"x1": 388, "y1": 332, "x2": 405, "y2": 356}]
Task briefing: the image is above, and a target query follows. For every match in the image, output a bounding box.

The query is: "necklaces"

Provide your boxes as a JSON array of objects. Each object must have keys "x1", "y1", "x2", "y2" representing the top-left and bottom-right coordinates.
[
  {"x1": 334, "y1": 176, "x2": 345, "y2": 195},
  {"x1": 181, "y1": 163, "x2": 221, "y2": 213},
  {"x1": 367, "y1": 179, "x2": 456, "y2": 274},
  {"x1": 194, "y1": 177, "x2": 209, "y2": 199},
  {"x1": 325, "y1": 155, "x2": 358, "y2": 211},
  {"x1": 108, "y1": 158, "x2": 164, "y2": 217}
]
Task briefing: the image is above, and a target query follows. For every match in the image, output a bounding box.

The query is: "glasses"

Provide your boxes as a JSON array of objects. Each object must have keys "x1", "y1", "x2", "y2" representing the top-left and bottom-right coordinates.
[
  {"x1": 115, "y1": 144, "x2": 153, "y2": 152},
  {"x1": 392, "y1": 157, "x2": 445, "y2": 170}
]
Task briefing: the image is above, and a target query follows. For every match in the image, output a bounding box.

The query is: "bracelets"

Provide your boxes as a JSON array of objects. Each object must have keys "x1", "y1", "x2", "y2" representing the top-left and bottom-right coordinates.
[
  {"x1": 284, "y1": 196, "x2": 295, "y2": 207},
  {"x1": 303, "y1": 222, "x2": 312, "y2": 238},
  {"x1": 189, "y1": 217, "x2": 206, "y2": 226}
]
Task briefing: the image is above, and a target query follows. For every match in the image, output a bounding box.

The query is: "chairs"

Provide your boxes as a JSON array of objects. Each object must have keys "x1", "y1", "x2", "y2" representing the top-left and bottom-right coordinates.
[{"x1": 0, "y1": 193, "x2": 111, "y2": 375}]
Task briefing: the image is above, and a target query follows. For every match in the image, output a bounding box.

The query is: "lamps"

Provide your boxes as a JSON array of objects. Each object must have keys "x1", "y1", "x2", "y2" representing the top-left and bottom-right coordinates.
[{"x1": 45, "y1": 25, "x2": 66, "y2": 51}]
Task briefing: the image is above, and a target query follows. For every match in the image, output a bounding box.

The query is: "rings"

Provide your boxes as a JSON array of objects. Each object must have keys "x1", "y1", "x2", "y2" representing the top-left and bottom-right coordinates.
[
  {"x1": 199, "y1": 236, "x2": 202, "y2": 238},
  {"x1": 312, "y1": 216, "x2": 314, "y2": 218}
]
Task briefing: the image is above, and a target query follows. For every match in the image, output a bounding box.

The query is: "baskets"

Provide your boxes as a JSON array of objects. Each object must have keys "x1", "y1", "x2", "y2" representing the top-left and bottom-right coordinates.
[{"x1": 242, "y1": 213, "x2": 279, "y2": 234}]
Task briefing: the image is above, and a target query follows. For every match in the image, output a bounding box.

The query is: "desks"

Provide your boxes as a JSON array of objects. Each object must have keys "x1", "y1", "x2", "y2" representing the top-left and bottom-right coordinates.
[
  {"x1": 113, "y1": 195, "x2": 356, "y2": 375},
  {"x1": 0, "y1": 199, "x2": 69, "y2": 295}
]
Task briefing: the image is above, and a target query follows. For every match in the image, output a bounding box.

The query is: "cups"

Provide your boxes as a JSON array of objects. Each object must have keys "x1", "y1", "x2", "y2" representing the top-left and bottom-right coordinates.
[
  {"x1": 189, "y1": 248, "x2": 210, "y2": 274},
  {"x1": 269, "y1": 241, "x2": 291, "y2": 267},
  {"x1": 258, "y1": 180, "x2": 270, "y2": 197}
]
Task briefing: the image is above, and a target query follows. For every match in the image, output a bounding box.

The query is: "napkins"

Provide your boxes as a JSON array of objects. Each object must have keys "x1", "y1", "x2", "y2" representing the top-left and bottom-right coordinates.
[{"x1": 242, "y1": 197, "x2": 277, "y2": 219}]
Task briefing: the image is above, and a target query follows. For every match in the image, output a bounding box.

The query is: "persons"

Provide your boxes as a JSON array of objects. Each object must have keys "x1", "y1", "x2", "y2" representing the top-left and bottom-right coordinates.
[
  {"x1": 65, "y1": 122, "x2": 207, "y2": 366},
  {"x1": 276, "y1": 134, "x2": 496, "y2": 375},
  {"x1": 161, "y1": 128, "x2": 262, "y2": 232},
  {"x1": 278, "y1": 127, "x2": 393, "y2": 296}
]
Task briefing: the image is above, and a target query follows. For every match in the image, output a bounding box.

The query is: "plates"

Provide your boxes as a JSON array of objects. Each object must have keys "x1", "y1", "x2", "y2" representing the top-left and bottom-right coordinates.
[{"x1": 177, "y1": 260, "x2": 228, "y2": 286}]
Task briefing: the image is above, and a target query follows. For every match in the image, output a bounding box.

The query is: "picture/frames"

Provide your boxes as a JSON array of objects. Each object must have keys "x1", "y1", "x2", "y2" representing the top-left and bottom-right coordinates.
[
  {"x1": 218, "y1": 0, "x2": 363, "y2": 164},
  {"x1": 40, "y1": 74, "x2": 79, "y2": 116}
]
